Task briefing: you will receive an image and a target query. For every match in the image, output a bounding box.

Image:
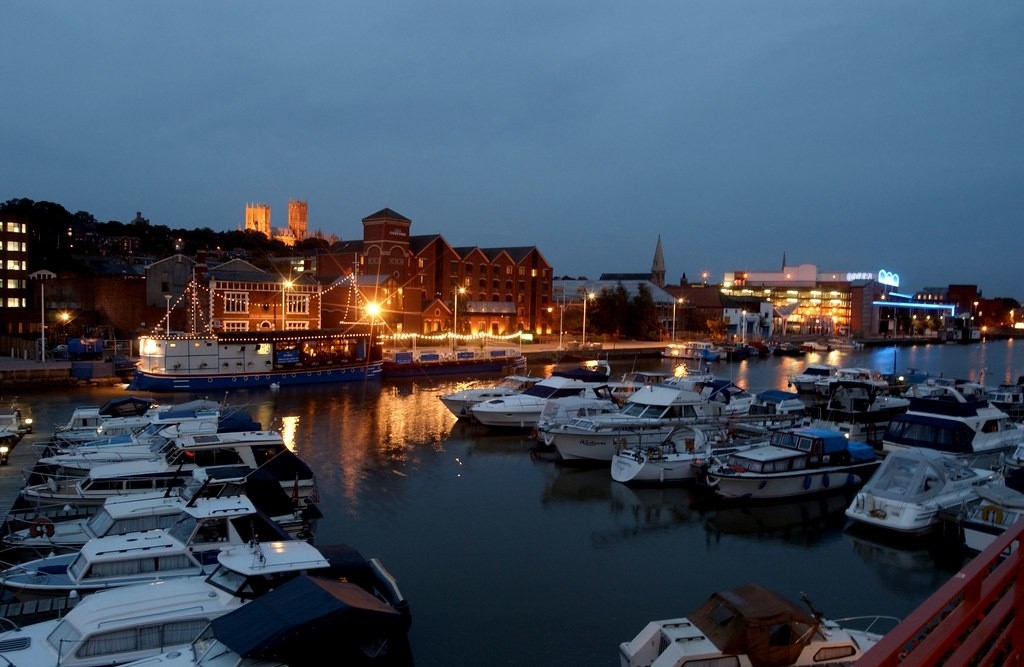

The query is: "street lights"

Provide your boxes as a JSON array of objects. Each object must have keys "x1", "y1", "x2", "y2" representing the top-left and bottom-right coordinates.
[
  {"x1": 742, "y1": 311, "x2": 746, "y2": 343},
  {"x1": 281, "y1": 281, "x2": 292, "y2": 349},
  {"x1": 672, "y1": 299, "x2": 683, "y2": 343},
  {"x1": 582, "y1": 292, "x2": 594, "y2": 349},
  {"x1": 453, "y1": 286, "x2": 465, "y2": 350},
  {"x1": 30, "y1": 269, "x2": 57, "y2": 363}
]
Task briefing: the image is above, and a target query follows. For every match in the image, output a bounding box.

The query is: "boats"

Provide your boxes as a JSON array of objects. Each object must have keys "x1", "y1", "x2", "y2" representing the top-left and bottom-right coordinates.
[
  {"x1": 125, "y1": 250, "x2": 385, "y2": 393},
  {"x1": 382, "y1": 332, "x2": 526, "y2": 376},
  {"x1": 440, "y1": 349, "x2": 1024, "y2": 566},
  {"x1": 656, "y1": 337, "x2": 866, "y2": 361},
  {"x1": 618, "y1": 582, "x2": 902, "y2": 667},
  {"x1": 0, "y1": 391, "x2": 413, "y2": 666}
]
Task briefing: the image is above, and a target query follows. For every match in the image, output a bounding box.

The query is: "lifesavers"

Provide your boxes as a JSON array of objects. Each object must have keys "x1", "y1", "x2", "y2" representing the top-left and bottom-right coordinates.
[
  {"x1": 531, "y1": 428, "x2": 539, "y2": 440},
  {"x1": 982, "y1": 504, "x2": 1003, "y2": 524},
  {"x1": 184, "y1": 450, "x2": 194, "y2": 457}
]
